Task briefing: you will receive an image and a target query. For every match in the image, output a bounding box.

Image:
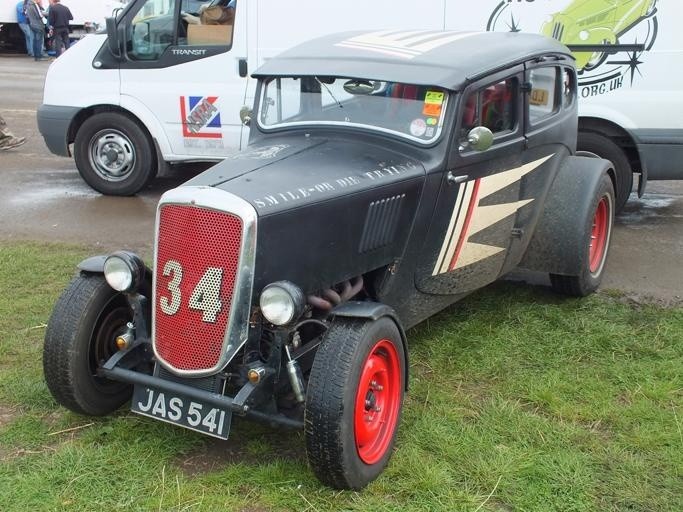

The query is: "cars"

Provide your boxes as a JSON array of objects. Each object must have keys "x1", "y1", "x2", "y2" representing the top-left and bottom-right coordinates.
[{"x1": 43, "y1": 28, "x2": 619, "y2": 491}]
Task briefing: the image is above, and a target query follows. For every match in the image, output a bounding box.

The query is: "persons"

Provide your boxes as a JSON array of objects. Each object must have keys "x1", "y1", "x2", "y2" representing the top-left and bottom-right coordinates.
[
  {"x1": 0, "y1": 116, "x2": 27, "y2": 151},
  {"x1": 182, "y1": 13, "x2": 201, "y2": 24},
  {"x1": 16, "y1": 0, "x2": 73, "y2": 61}
]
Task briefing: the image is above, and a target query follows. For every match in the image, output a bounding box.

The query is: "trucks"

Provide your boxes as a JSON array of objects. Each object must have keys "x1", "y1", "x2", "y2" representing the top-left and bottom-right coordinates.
[{"x1": 37, "y1": 1, "x2": 682, "y2": 216}]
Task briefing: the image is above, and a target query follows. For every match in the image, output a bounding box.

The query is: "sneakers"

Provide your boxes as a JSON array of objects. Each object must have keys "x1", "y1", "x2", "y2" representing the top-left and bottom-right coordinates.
[
  {"x1": 0, "y1": 137, "x2": 26, "y2": 149},
  {"x1": 36, "y1": 56, "x2": 49, "y2": 60}
]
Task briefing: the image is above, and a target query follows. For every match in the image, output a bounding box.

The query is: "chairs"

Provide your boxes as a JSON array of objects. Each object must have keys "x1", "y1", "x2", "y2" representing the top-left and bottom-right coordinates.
[{"x1": 381, "y1": 81, "x2": 514, "y2": 136}]
